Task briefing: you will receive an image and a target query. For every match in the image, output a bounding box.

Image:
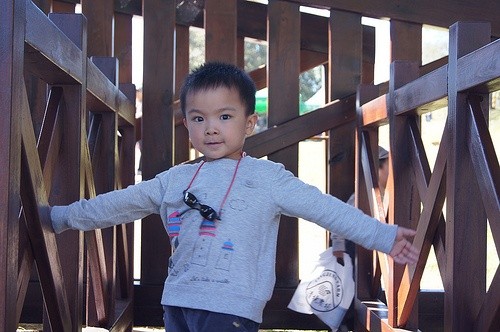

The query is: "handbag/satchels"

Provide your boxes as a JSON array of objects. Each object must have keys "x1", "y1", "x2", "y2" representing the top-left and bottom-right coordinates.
[{"x1": 287, "y1": 247, "x2": 356, "y2": 331}]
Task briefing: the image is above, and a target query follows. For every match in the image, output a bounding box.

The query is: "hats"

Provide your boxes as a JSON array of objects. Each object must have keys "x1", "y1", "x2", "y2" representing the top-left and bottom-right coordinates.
[{"x1": 378, "y1": 145, "x2": 389, "y2": 160}]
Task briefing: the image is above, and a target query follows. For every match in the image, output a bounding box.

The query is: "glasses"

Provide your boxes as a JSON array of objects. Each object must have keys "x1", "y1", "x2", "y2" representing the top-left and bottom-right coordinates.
[{"x1": 183, "y1": 189, "x2": 222, "y2": 222}]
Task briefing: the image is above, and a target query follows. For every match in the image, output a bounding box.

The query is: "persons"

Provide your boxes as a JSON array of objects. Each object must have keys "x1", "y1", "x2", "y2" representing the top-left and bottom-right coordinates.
[
  {"x1": 330, "y1": 146, "x2": 389, "y2": 332},
  {"x1": 50, "y1": 60, "x2": 420, "y2": 332}
]
